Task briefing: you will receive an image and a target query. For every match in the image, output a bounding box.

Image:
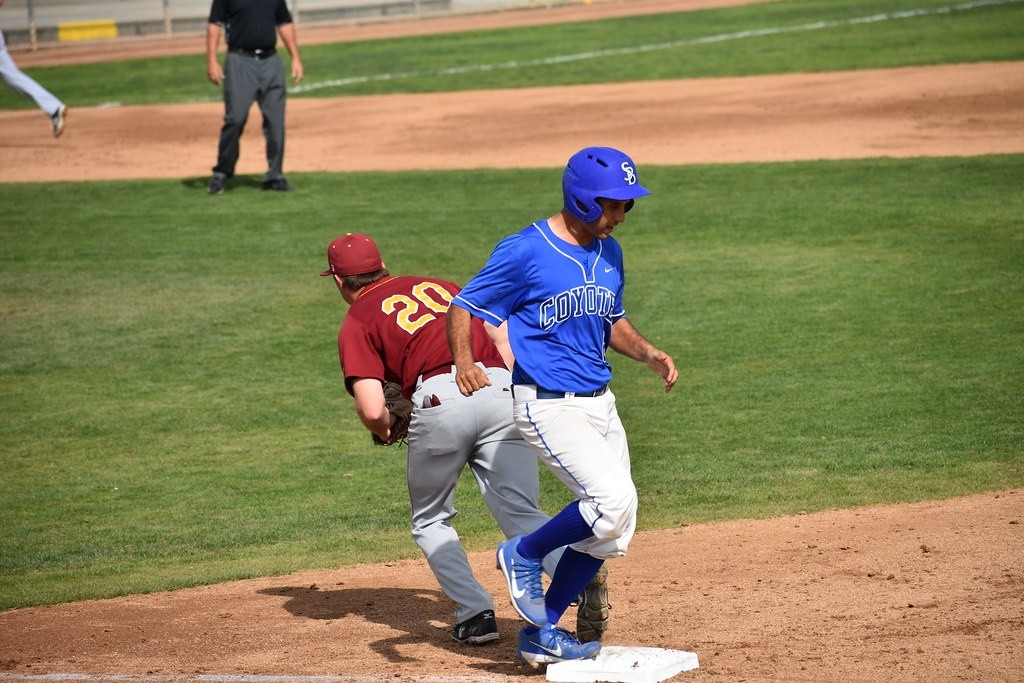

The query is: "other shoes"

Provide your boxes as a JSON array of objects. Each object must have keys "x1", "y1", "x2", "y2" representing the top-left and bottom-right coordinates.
[
  {"x1": 52, "y1": 103, "x2": 67, "y2": 138},
  {"x1": 262, "y1": 178, "x2": 296, "y2": 193},
  {"x1": 204, "y1": 170, "x2": 227, "y2": 197}
]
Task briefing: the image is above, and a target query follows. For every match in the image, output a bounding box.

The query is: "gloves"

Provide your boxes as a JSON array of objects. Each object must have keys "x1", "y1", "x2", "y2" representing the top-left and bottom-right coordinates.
[{"x1": 422, "y1": 394, "x2": 441, "y2": 408}]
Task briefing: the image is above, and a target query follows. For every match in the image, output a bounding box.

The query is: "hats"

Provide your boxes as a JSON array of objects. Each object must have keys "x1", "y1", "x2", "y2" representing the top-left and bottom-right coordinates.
[{"x1": 319, "y1": 232, "x2": 384, "y2": 277}]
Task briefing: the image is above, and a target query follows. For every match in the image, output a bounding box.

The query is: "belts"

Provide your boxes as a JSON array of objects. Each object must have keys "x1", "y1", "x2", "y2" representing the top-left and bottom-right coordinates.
[
  {"x1": 408, "y1": 360, "x2": 510, "y2": 404},
  {"x1": 227, "y1": 45, "x2": 278, "y2": 62},
  {"x1": 510, "y1": 384, "x2": 609, "y2": 400}
]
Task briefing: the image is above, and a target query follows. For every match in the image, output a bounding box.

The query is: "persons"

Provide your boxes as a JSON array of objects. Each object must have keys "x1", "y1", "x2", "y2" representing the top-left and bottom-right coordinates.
[
  {"x1": 445, "y1": 146, "x2": 678, "y2": 668},
  {"x1": 206, "y1": 0, "x2": 303, "y2": 194},
  {"x1": 0, "y1": 31, "x2": 68, "y2": 139},
  {"x1": 320, "y1": 234, "x2": 608, "y2": 648}
]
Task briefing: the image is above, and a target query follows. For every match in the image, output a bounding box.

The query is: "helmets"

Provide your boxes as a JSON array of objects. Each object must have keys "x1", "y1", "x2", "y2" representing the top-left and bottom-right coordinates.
[{"x1": 562, "y1": 146, "x2": 653, "y2": 224}]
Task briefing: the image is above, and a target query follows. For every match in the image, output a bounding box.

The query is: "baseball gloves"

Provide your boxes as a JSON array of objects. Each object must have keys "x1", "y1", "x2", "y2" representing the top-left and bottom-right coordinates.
[{"x1": 371, "y1": 381, "x2": 414, "y2": 448}]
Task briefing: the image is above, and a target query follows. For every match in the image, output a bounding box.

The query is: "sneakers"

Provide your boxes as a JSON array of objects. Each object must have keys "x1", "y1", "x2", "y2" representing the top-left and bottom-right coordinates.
[
  {"x1": 516, "y1": 624, "x2": 602, "y2": 667},
  {"x1": 450, "y1": 609, "x2": 500, "y2": 644},
  {"x1": 497, "y1": 536, "x2": 548, "y2": 628},
  {"x1": 576, "y1": 565, "x2": 611, "y2": 646}
]
